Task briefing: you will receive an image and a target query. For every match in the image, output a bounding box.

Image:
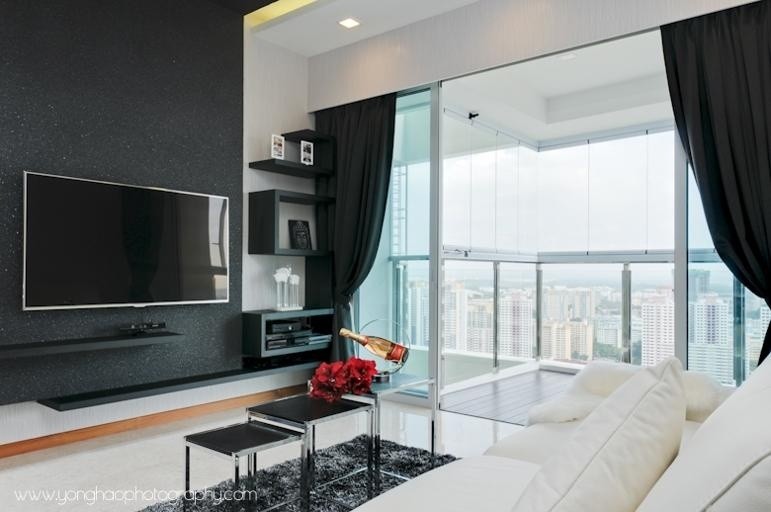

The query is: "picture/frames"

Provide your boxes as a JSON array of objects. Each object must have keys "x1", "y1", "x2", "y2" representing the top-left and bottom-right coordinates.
[
  {"x1": 288, "y1": 219, "x2": 312, "y2": 250},
  {"x1": 300, "y1": 140, "x2": 315, "y2": 165},
  {"x1": 271, "y1": 134, "x2": 285, "y2": 160}
]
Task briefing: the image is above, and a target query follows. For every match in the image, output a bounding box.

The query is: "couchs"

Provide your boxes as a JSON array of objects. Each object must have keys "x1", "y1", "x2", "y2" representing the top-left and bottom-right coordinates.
[{"x1": 350, "y1": 345, "x2": 770, "y2": 511}]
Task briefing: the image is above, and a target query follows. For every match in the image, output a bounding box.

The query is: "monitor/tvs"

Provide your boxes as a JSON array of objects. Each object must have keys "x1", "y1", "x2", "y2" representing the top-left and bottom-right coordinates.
[{"x1": 21, "y1": 170, "x2": 230, "y2": 310}]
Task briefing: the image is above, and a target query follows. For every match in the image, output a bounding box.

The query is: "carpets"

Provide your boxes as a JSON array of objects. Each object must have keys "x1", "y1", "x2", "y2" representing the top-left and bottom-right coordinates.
[{"x1": 140, "y1": 433, "x2": 461, "y2": 512}]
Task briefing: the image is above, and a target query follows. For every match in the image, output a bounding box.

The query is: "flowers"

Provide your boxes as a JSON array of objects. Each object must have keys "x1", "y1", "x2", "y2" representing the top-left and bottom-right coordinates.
[{"x1": 306, "y1": 357, "x2": 377, "y2": 403}]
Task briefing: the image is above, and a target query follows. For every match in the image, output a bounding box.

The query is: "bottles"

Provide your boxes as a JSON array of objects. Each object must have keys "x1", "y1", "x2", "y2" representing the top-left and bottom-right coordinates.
[
  {"x1": 273, "y1": 268, "x2": 290, "y2": 308},
  {"x1": 290, "y1": 274, "x2": 300, "y2": 308},
  {"x1": 338, "y1": 327, "x2": 410, "y2": 364}
]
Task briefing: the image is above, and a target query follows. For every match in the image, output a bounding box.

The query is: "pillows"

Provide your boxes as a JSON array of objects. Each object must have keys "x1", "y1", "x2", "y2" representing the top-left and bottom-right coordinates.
[{"x1": 514, "y1": 356, "x2": 687, "y2": 510}]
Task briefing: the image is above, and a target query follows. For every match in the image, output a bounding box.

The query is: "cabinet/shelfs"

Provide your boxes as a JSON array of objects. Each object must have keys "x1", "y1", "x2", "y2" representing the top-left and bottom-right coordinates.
[{"x1": 1, "y1": 128, "x2": 336, "y2": 412}]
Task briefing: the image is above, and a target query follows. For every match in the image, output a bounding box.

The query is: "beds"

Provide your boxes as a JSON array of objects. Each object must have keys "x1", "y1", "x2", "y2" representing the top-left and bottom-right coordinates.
[{"x1": 182, "y1": 421, "x2": 300, "y2": 512}]
